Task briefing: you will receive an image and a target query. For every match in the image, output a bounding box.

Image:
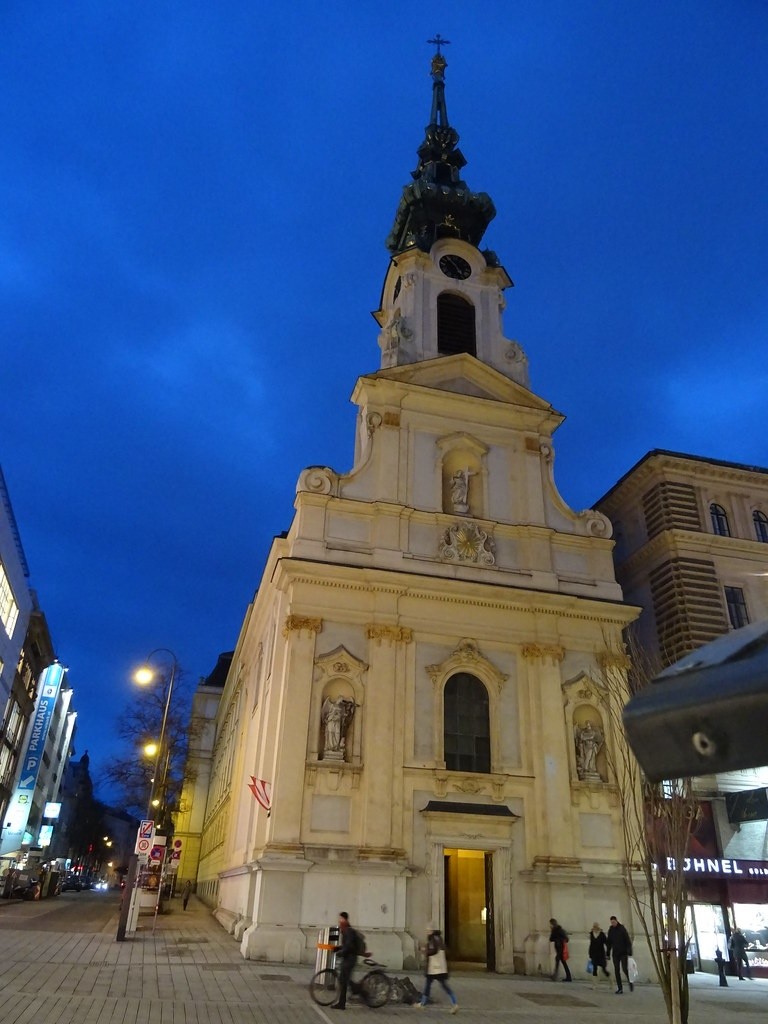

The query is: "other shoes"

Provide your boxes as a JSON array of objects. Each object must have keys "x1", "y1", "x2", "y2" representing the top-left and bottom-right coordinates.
[
  {"x1": 414, "y1": 1002, "x2": 426, "y2": 1011},
  {"x1": 550, "y1": 976, "x2": 556, "y2": 981},
  {"x1": 750, "y1": 977, "x2": 753, "y2": 980},
  {"x1": 739, "y1": 978, "x2": 745, "y2": 980},
  {"x1": 631, "y1": 984, "x2": 634, "y2": 991},
  {"x1": 616, "y1": 989, "x2": 623, "y2": 993},
  {"x1": 562, "y1": 978, "x2": 572, "y2": 982},
  {"x1": 450, "y1": 1005, "x2": 459, "y2": 1015},
  {"x1": 331, "y1": 1003, "x2": 345, "y2": 1010}
]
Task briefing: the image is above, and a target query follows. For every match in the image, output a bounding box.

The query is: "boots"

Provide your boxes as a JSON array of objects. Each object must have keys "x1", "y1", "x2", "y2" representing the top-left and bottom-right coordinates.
[
  {"x1": 590, "y1": 975, "x2": 597, "y2": 989},
  {"x1": 608, "y1": 974, "x2": 614, "y2": 989}
]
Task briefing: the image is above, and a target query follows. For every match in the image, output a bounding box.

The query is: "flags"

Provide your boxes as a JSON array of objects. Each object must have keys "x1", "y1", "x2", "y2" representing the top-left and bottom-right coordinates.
[{"x1": 247, "y1": 776, "x2": 271, "y2": 811}]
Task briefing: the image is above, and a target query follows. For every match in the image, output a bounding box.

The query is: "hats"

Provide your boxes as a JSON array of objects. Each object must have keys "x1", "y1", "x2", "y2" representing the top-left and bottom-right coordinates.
[
  {"x1": 341, "y1": 912, "x2": 348, "y2": 919},
  {"x1": 425, "y1": 920, "x2": 439, "y2": 931},
  {"x1": 590, "y1": 922, "x2": 599, "y2": 931}
]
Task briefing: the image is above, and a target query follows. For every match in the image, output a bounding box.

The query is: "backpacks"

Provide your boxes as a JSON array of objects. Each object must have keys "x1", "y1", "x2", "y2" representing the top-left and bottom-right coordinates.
[{"x1": 345, "y1": 929, "x2": 365, "y2": 953}]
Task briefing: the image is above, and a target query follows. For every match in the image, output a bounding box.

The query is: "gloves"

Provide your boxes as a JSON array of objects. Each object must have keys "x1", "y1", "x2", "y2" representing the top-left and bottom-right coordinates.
[
  {"x1": 333, "y1": 947, "x2": 340, "y2": 952},
  {"x1": 336, "y1": 952, "x2": 342, "y2": 957}
]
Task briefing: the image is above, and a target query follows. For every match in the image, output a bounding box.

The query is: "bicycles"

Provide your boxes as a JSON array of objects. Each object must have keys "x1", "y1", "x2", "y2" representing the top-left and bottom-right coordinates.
[{"x1": 309, "y1": 944, "x2": 392, "y2": 1009}]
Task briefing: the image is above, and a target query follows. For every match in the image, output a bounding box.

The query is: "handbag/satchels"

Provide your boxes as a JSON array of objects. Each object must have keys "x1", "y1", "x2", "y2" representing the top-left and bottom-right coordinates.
[
  {"x1": 427, "y1": 935, "x2": 448, "y2": 975},
  {"x1": 627, "y1": 956, "x2": 639, "y2": 983},
  {"x1": 562, "y1": 942, "x2": 568, "y2": 959},
  {"x1": 586, "y1": 961, "x2": 593, "y2": 973}
]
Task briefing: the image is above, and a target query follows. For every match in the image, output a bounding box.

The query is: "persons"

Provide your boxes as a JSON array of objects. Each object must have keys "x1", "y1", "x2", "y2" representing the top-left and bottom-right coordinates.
[
  {"x1": 587, "y1": 922, "x2": 613, "y2": 990},
  {"x1": 548, "y1": 919, "x2": 572, "y2": 982},
  {"x1": 574, "y1": 720, "x2": 604, "y2": 772},
  {"x1": 329, "y1": 912, "x2": 361, "y2": 1009},
  {"x1": 413, "y1": 921, "x2": 460, "y2": 1013},
  {"x1": 605, "y1": 916, "x2": 634, "y2": 994},
  {"x1": 324, "y1": 695, "x2": 346, "y2": 750},
  {"x1": 730, "y1": 928, "x2": 755, "y2": 980},
  {"x1": 180, "y1": 880, "x2": 191, "y2": 911}
]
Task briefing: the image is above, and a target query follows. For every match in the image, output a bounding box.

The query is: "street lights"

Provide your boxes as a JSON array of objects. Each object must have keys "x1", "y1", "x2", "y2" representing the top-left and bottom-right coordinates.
[{"x1": 115, "y1": 646, "x2": 180, "y2": 942}]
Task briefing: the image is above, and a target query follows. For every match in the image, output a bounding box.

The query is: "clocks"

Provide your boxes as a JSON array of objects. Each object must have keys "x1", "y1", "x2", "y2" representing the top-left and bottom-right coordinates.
[{"x1": 439, "y1": 254, "x2": 472, "y2": 280}]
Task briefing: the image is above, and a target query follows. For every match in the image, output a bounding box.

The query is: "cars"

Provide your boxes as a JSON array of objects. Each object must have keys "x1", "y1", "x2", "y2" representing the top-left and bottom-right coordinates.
[
  {"x1": 61, "y1": 875, "x2": 81, "y2": 892},
  {"x1": 77, "y1": 874, "x2": 108, "y2": 890}
]
